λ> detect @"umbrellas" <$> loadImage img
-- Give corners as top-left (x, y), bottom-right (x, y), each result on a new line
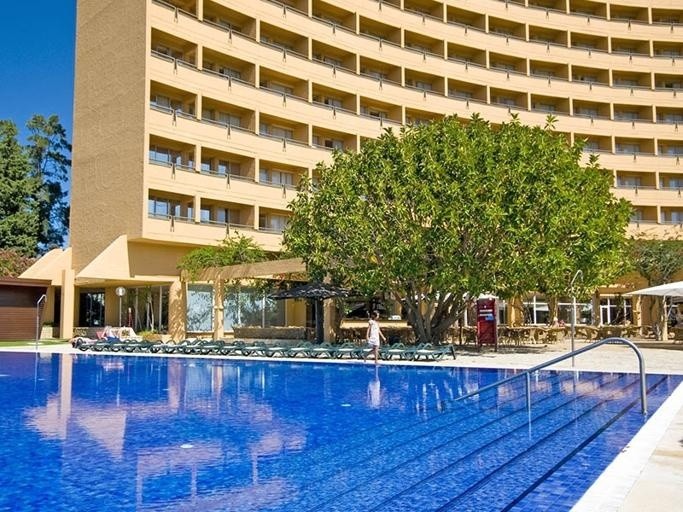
top-left (626, 281), bottom-right (683, 297)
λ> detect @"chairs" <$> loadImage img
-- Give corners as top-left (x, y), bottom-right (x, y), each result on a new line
top-left (457, 321), bottom-right (683, 349)
top-left (75, 336), bottom-right (457, 362)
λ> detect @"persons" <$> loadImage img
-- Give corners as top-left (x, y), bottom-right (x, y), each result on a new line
top-left (364, 310), bottom-right (387, 363)
top-left (366, 365), bottom-right (385, 416)
